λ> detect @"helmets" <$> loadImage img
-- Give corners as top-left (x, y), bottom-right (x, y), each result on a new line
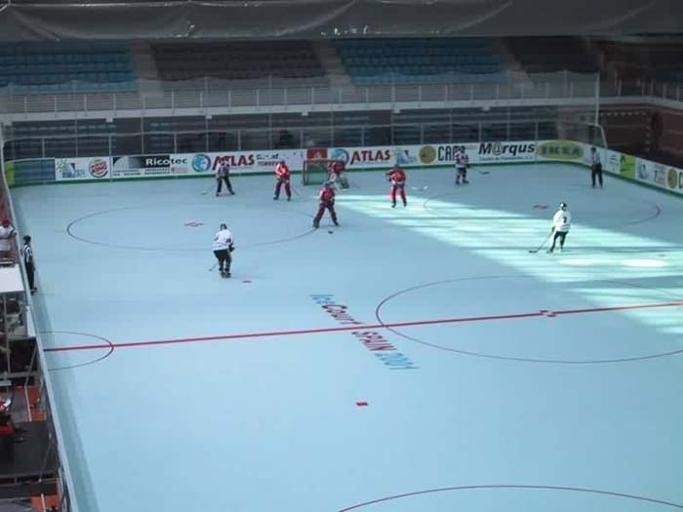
top-left (560, 203), bottom-right (569, 210)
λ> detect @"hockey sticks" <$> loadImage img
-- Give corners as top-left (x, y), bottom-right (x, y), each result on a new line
top-left (529, 232), bottom-right (553, 253)
top-left (208, 262), bottom-right (219, 272)
top-left (471, 167), bottom-right (490, 175)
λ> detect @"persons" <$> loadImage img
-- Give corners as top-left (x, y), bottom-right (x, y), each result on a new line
top-left (546, 202), bottom-right (571, 253)
top-left (213, 223), bottom-right (233, 277)
top-left (386, 163), bottom-right (408, 210)
top-left (588, 146), bottom-right (604, 189)
top-left (0, 217), bottom-right (16, 263)
top-left (214, 157), bottom-right (235, 198)
top-left (326, 160), bottom-right (348, 188)
top-left (0, 398), bottom-right (29, 446)
top-left (273, 159), bottom-right (293, 200)
top-left (20, 233), bottom-right (38, 298)
top-left (312, 179), bottom-right (338, 228)
top-left (450, 144), bottom-right (470, 185)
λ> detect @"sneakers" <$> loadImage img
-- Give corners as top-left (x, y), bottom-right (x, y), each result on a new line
top-left (221, 272), bottom-right (230, 278)
top-left (216, 191), bottom-right (235, 197)
top-left (455, 180), bottom-right (469, 184)
top-left (313, 221), bottom-right (338, 228)
top-left (391, 203), bottom-right (406, 207)
top-left (273, 197), bottom-right (290, 201)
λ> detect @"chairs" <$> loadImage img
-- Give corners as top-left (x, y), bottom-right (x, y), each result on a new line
top-left (1, 34), bottom-right (683, 161)
